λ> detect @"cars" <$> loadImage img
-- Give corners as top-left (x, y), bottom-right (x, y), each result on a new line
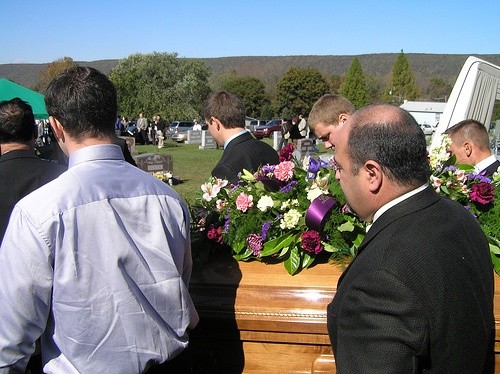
top-left (164, 121), bottom-right (193, 142)
top-left (249, 118), bottom-right (290, 140)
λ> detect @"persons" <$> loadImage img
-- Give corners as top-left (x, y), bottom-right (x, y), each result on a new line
top-left (327, 105), bottom-right (495, 374)
top-left (308, 94), bottom-right (356, 152)
top-left (442, 119), bottom-right (500, 180)
top-left (0, 90), bottom-right (308, 246)
top-left (0, 66), bottom-right (199, 374)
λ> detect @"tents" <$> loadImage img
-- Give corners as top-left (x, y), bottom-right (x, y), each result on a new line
top-left (0, 78), bottom-right (49, 119)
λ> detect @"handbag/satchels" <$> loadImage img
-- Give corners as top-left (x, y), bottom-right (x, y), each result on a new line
top-left (284, 132), bottom-right (291, 139)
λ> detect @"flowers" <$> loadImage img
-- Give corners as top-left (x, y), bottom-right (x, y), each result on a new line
top-left (186, 133), bottom-right (500, 276)
top-left (153, 171), bottom-right (172, 181)
